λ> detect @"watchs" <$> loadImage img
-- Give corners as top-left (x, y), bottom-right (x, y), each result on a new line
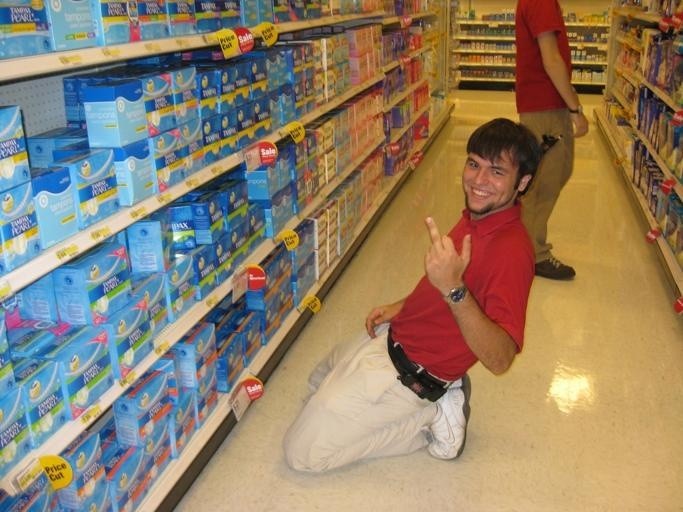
top-left (438, 284), bottom-right (471, 306)
top-left (569, 103), bottom-right (586, 115)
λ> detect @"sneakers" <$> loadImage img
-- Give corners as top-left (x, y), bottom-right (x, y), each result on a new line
top-left (429, 379), bottom-right (467, 462)
top-left (534, 254), bottom-right (576, 282)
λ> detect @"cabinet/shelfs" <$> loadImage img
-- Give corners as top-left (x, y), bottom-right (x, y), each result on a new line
top-left (0, 9), bottom-right (455, 512)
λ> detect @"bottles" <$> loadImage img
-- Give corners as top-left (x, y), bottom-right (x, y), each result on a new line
top-left (452, 8), bottom-right (516, 78)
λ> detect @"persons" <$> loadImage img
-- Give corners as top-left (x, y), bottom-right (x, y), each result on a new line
top-left (282, 119), bottom-right (541, 473)
top-left (514, 0), bottom-right (589, 281)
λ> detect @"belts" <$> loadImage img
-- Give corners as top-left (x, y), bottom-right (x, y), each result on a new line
top-left (387, 329), bottom-right (446, 402)
